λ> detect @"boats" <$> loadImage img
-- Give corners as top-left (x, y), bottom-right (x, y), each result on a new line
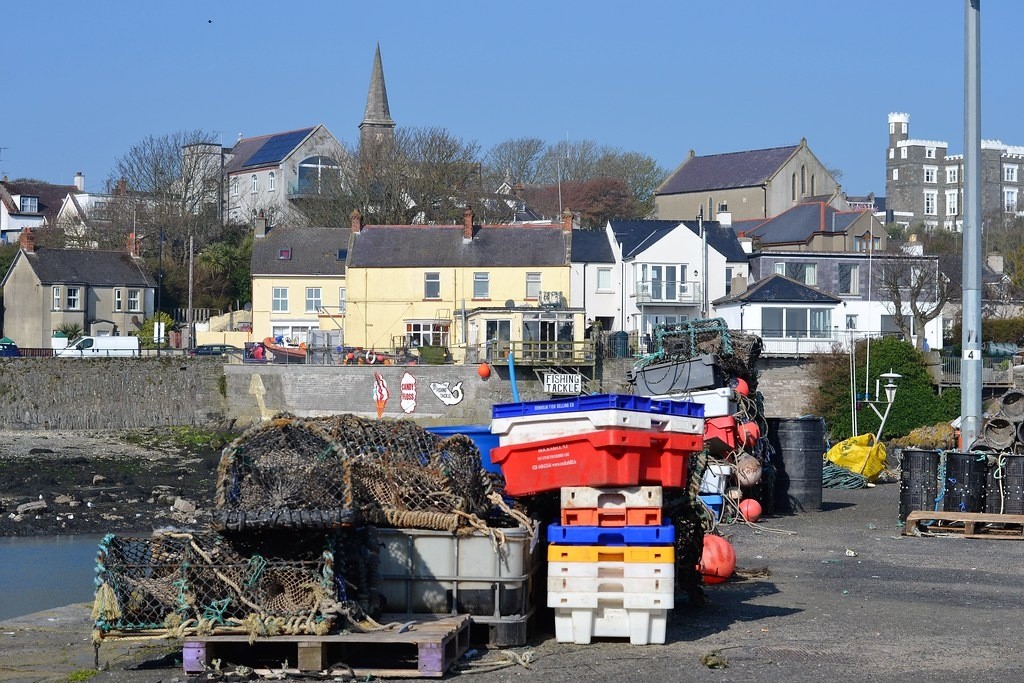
top-left (264, 334), bottom-right (308, 359)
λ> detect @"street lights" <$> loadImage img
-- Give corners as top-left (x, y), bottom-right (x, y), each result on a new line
top-left (157, 226), bottom-right (168, 357)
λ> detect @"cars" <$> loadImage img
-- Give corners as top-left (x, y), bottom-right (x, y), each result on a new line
top-left (189, 343), bottom-right (243, 356)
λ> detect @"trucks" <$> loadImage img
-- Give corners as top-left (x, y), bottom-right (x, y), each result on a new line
top-left (56, 336), bottom-right (141, 358)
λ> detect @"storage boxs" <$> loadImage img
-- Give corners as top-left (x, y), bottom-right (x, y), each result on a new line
top-left (627, 353), bottom-right (746, 527)
top-left (490, 394), bottom-right (704, 646)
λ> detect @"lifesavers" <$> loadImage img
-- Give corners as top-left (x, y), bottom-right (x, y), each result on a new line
top-left (366, 351), bottom-right (376, 364)
